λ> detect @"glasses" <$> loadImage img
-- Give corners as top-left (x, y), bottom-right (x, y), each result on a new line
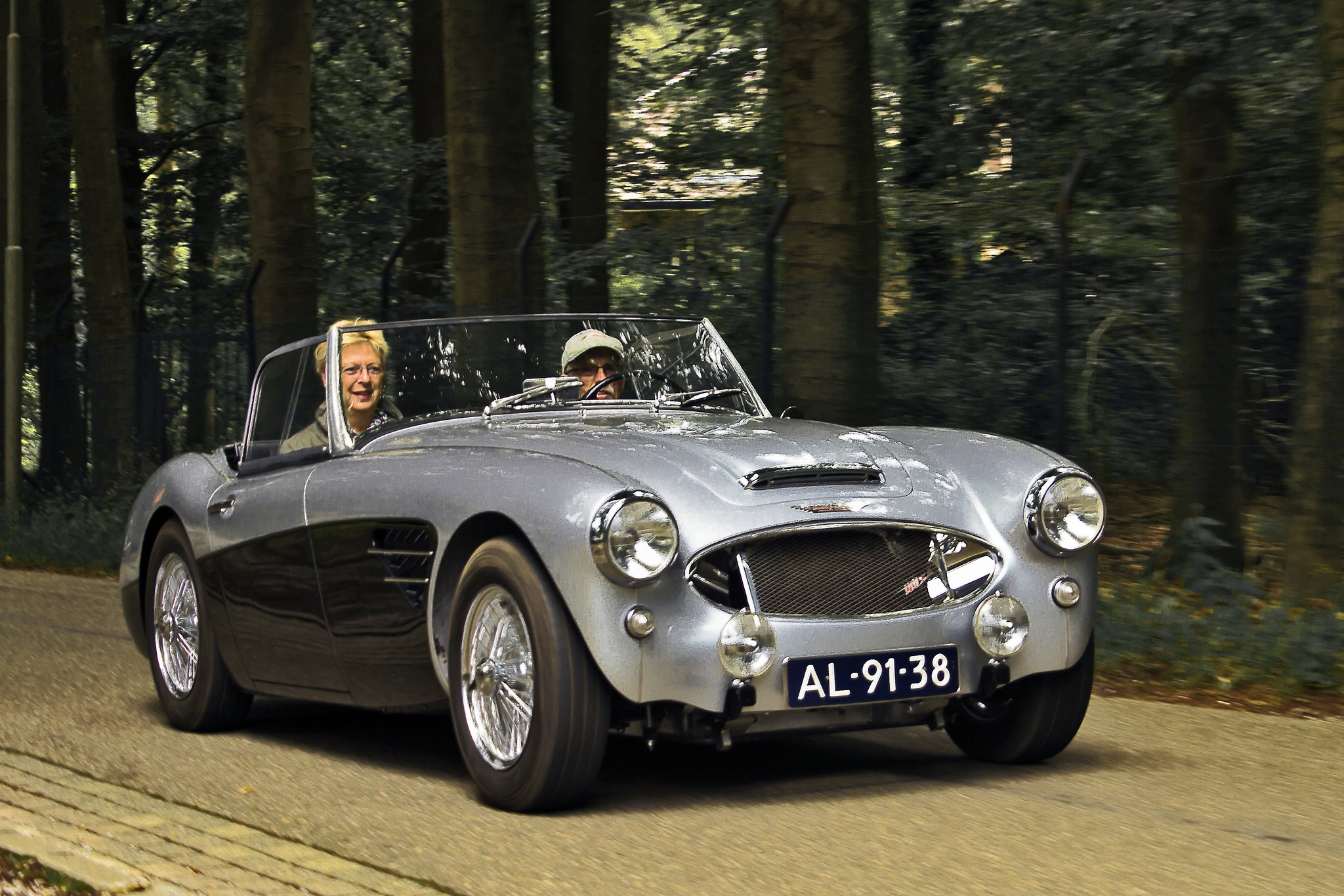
top-left (564, 363), bottom-right (624, 376)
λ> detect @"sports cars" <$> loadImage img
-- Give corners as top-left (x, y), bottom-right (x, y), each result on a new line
top-left (118, 312), bottom-right (1108, 815)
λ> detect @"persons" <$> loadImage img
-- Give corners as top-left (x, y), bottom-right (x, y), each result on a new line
top-left (560, 329), bottom-right (626, 399)
top-left (280, 319), bottom-right (403, 454)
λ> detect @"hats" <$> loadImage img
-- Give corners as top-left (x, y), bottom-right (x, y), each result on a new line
top-left (562, 329), bottom-right (625, 374)
